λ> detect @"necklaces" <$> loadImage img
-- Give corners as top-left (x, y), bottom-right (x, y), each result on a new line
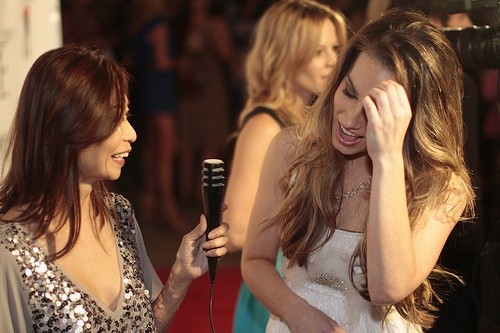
top-left (335, 176), bottom-right (372, 199)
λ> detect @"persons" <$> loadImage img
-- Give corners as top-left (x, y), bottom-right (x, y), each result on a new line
top-left (130, 16), bottom-right (192, 233)
top-left (0, 46), bottom-right (230, 333)
top-left (240, 9), bottom-right (475, 333)
top-left (365, 0), bottom-right (472, 28)
top-left (219, 0), bottom-right (350, 333)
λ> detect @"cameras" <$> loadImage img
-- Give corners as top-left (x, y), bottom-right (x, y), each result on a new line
top-left (442, 24), bottom-right (499, 68)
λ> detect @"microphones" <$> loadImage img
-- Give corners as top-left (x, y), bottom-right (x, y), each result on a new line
top-left (202, 159), bottom-right (226, 279)
